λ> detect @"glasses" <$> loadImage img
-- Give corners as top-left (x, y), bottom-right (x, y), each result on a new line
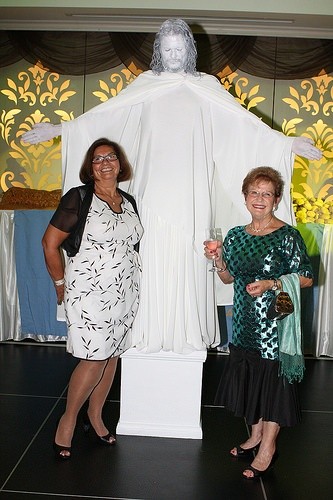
top-left (91, 154), bottom-right (120, 164)
top-left (246, 189), bottom-right (276, 198)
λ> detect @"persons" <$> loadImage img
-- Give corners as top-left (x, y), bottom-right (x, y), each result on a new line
top-left (203, 167), bottom-right (314, 478)
top-left (21, 19), bottom-right (323, 348)
top-left (41, 138), bottom-right (144, 458)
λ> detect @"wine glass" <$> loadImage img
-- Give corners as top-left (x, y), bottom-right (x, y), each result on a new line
top-left (206, 228), bottom-right (222, 272)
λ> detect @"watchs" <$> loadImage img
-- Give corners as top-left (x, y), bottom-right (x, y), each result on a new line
top-left (271, 280), bottom-right (278, 290)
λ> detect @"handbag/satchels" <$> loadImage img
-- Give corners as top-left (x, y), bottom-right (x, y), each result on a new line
top-left (267, 278), bottom-right (294, 320)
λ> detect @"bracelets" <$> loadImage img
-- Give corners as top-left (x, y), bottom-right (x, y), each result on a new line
top-left (216, 262), bottom-right (227, 272)
top-left (54, 278), bottom-right (66, 285)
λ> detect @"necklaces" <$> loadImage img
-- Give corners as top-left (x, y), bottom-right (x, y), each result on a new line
top-left (102, 191), bottom-right (120, 205)
top-left (250, 217), bottom-right (273, 233)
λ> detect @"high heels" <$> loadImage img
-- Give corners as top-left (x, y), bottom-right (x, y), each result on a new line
top-left (53, 415), bottom-right (76, 460)
top-left (228, 437), bottom-right (262, 458)
top-left (82, 411), bottom-right (117, 447)
top-left (240, 445), bottom-right (281, 479)
